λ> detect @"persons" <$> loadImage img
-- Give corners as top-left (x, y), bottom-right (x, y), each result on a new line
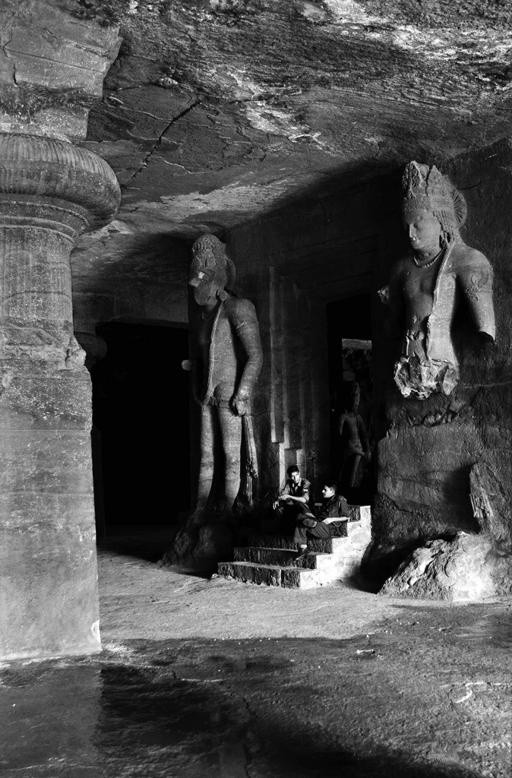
top-left (272, 465), bottom-right (310, 514)
top-left (293, 480), bottom-right (350, 560)
top-left (374, 160), bottom-right (496, 398)
top-left (338, 383), bottom-right (371, 488)
top-left (185, 234), bottom-right (262, 522)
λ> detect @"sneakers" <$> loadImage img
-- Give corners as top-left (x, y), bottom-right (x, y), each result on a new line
top-left (295, 547), bottom-right (311, 561)
top-left (303, 517), bottom-right (317, 528)
